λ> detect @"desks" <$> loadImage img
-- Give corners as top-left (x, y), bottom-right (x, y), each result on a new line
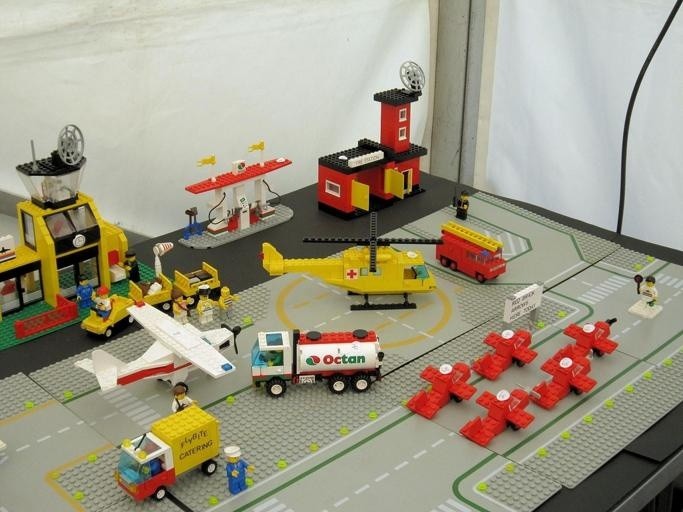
top-left (0, 146), bottom-right (683, 512)
